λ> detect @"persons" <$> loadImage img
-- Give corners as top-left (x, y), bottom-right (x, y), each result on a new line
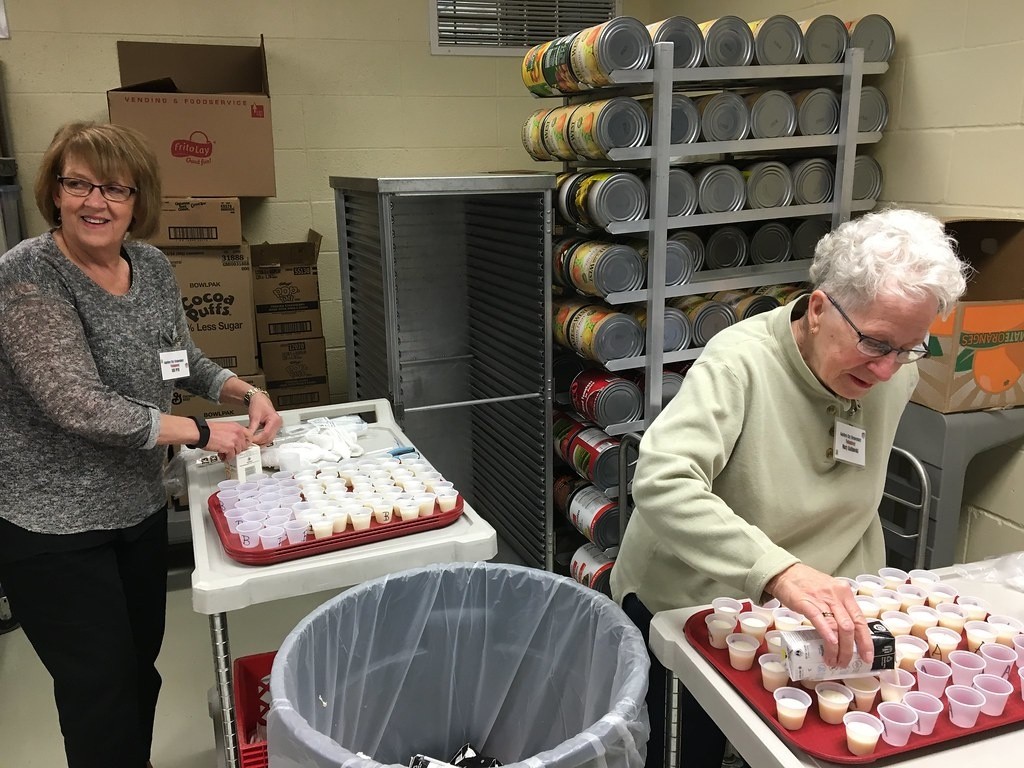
top-left (0, 119), bottom-right (284, 768)
top-left (608, 206), bottom-right (976, 768)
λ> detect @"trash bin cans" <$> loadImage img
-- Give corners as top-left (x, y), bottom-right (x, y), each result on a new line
top-left (267, 561), bottom-right (655, 768)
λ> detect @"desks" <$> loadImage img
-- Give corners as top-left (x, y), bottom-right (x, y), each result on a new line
top-left (649, 556), bottom-right (1022, 768)
top-left (188, 404), bottom-right (497, 768)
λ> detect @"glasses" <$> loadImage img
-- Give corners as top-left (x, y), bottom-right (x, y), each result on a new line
top-left (56, 172), bottom-right (139, 203)
top-left (818, 288), bottom-right (931, 364)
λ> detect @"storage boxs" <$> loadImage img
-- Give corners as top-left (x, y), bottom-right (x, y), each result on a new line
top-left (104, 35), bottom-right (331, 512)
top-left (908, 216), bottom-right (1024, 414)
top-left (232, 649), bottom-right (278, 768)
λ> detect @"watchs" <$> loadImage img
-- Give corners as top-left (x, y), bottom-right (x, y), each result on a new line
top-left (244, 386), bottom-right (271, 406)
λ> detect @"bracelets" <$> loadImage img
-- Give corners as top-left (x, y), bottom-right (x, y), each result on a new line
top-left (185, 415), bottom-right (211, 449)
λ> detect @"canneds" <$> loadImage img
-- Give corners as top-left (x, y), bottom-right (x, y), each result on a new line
top-left (520, 13), bottom-right (897, 598)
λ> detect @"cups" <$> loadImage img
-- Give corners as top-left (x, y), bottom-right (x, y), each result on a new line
top-left (925, 626), bottom-right (963, 663)
top-left (948, 649), bottom-right (986, 687)
top-left (1013, 634), bottom-right (1024, 668)
top-left (980, 643), bottom-right (1019, 682)
top-left (773, 687), bottom-right (812, 730)
top-left (943, 685), bottom-right (986, 728)
top-left (844, 709), bottom-right (884, 757)
top-left (1018, 667), bottom-right (1024, 701)
top-left (903, 691), bottom-right (944, 736)
top-left (758, 653), bottom-right (790, 692)
top-left (988, 615), bottom-right (1024, 650)
top-left (217, 471), bottom-right (311, 549)
top-left (815, 681), bottom-right (854, 724)
top-left (964, 620), bottom-right (999, 656)
top-left (835, 568), bottom-right (989, 643)
top-left (972, 673), bottom-right (1013, 716)
top-left (842, 677), bottom-right (882, 713)
top-left (295, 453), bottom-right (459, 539)
top-left (877, 701), bottom-right (919, 748)
top-left (914, 658), bottom-right (952, 698)
top-left (880, 667), bottom-right (917, 704)
top-left (705, 597), bottom-right (815, 671)
top-left (895, 635), bottom-right (930, 673)
top-left (801, 680), bottom-right (822, 690)
